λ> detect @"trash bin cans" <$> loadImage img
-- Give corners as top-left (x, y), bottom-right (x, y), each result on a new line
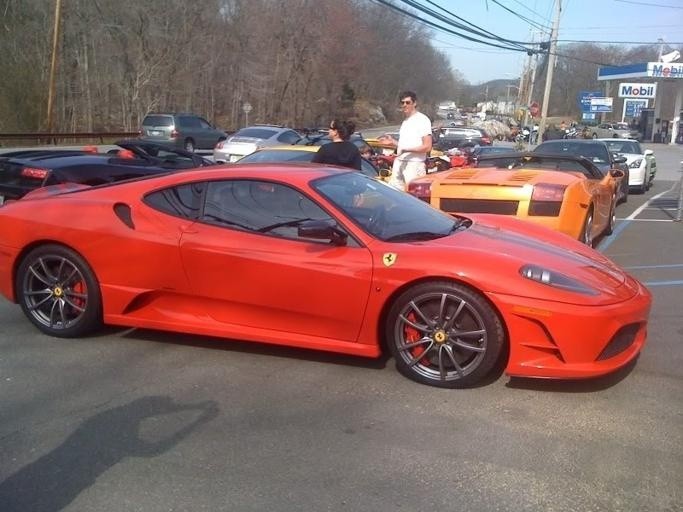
top-left (654, 134), bottom-right (662, 142)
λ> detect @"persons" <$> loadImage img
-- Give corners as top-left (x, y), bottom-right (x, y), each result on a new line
top-left (310, 117), bottom-right (361, 170)
top-left (358, 145), bottom-right (373, 158)
top-left (385, 91), bottom-right (433, 196)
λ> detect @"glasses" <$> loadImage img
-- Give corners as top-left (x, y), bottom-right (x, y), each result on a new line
top-left (401, 101), bottom-right (411, 104)
top-left (329, 126), bottom-right (335, 130)
